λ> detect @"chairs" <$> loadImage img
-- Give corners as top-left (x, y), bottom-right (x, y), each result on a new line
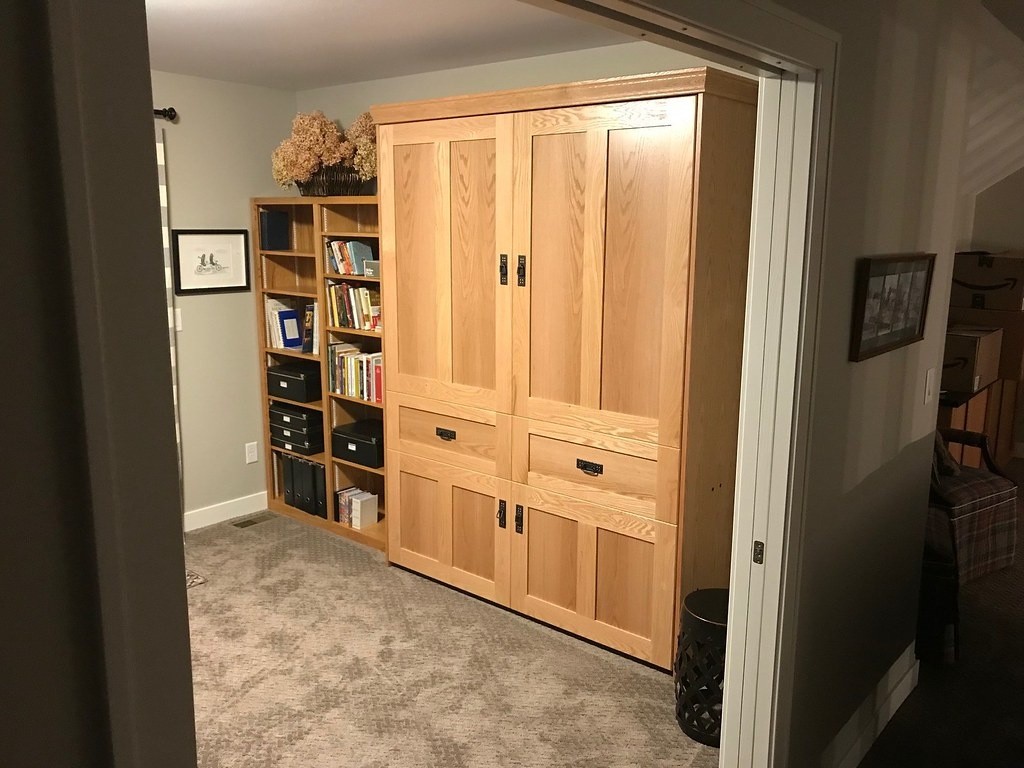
top-left (924, 402), bottom-right (1017, 583)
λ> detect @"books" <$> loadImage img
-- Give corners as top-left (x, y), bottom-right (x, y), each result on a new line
top-left (260, 212), bottom-right (289, 250)
top-left (327, 340), bottom-right (383, 403)
top-left (334, 486), bottom-right (378, 529)
top-left (265, 294), bottom-right (319, 355)
top-left (326, 282), bottom-right (380, 331)
top-left (326, 240), bottom-right (373, 276)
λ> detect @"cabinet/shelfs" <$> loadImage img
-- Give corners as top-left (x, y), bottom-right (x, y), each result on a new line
top-left (249, 195), bottom-right (387, 552)
top-left (369, 67), bottom-right (757, 675)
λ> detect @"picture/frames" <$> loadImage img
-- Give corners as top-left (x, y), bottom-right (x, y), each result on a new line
top-left (850, 252), bottom-right (939, 363)
top-left (172, 228), bottom-right (251, 297)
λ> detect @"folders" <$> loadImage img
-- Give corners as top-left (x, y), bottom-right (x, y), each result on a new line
top-left (282, 453), bottom-right (294, 507)
top-left (315, 464), bottom-right (327, 520)
top-left (292, 457), bottom-right (303, 511)
top-left (302, 460), bottom-right (316, 516)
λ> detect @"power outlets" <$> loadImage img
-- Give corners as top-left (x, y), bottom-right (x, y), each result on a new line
top-left (245, 442), bottom-right (258, 464)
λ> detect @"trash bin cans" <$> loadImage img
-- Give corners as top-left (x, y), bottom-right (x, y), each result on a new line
top-left (673, 587), bottom-right (730, 747)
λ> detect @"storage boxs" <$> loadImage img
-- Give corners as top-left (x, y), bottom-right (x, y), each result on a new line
top-left (267, 363), bottom-right (321, 404)
top-left (333, 419), bottom-right (384, 469)
top-left (941, 248), bottom-right (1024, 393)
top-left (268, 404), bottom-right (325, 518)
